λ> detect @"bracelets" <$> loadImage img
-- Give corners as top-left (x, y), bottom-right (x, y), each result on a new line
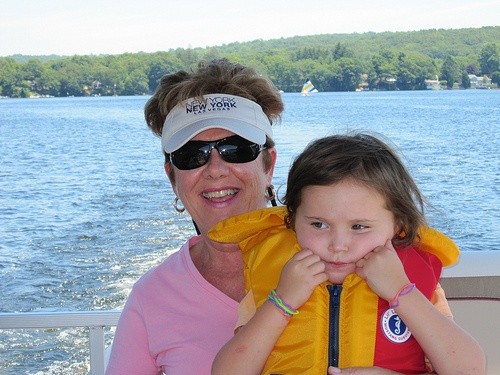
top-left (387, 282), bottom-right (417, 309)
top-left (263, 288), bottom-right (304, 322)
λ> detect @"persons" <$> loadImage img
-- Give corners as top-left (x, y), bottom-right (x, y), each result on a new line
top-left (207, 135), bottom-right (486, 375)
top-left (106, 58), bottom-right (284, 375)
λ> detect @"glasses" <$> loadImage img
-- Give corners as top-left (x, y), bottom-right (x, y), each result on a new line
top-left (168, 135), bottom-right (261, 171)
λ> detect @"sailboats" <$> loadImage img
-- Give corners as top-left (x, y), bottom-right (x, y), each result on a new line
top-left (301, 78), bottom-right (319, 95)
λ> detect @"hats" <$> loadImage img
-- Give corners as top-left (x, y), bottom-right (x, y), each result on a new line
top-left (162, 94), bottom-right (273, 154)
